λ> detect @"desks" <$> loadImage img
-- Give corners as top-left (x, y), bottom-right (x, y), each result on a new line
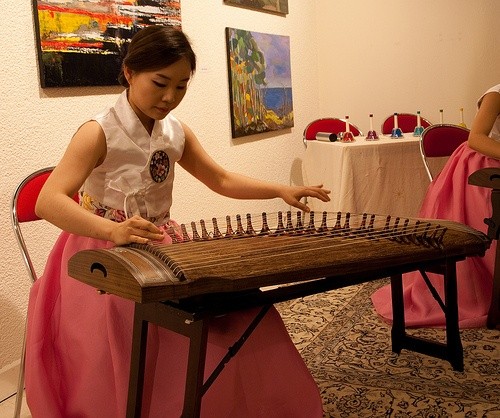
top-left (302, 132), bottom-right (452, 221)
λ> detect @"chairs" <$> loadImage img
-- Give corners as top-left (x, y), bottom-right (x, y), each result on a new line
top-left (302, 116), bottom-right (363, 148)
top-left (11, 165), bottom-right (80, 418)
top-left (420, 123), bottom-right (471, 181)
top-left (381, 112), bottom-right (433, 134)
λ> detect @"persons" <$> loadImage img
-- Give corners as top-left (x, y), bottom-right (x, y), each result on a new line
top-left (25, 24), bottom-right (330, 418)
top-left (371, 83), bottom-right (500, 330)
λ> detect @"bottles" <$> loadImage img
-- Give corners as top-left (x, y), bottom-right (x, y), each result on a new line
top-left (316, 132), bottom-right (337, 142)
